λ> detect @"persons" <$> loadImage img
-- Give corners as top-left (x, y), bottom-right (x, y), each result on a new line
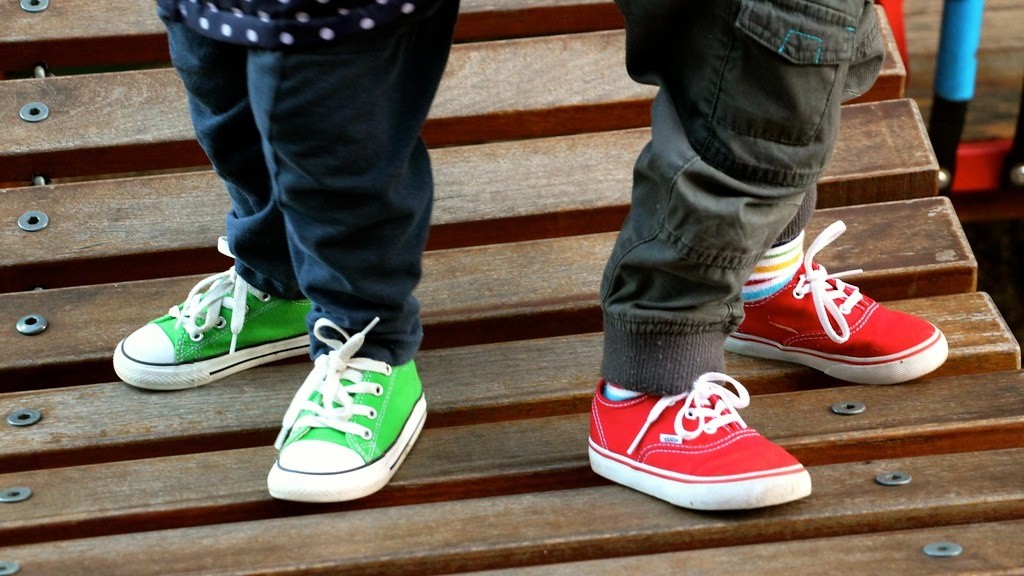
top-left (113, 0), bottom-right (463, 503)
top-left (588, 0), bottom-right (949, 511)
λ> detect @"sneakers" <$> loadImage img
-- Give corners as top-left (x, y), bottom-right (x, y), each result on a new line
top-left (113, 236), bottom-right (313, 390)
top-left (588, 371), bottom-right (812, 511)
top-left (724, 220), bottom-right (949, 384)
top-left (266, 317), bottom-right (427, 502)
